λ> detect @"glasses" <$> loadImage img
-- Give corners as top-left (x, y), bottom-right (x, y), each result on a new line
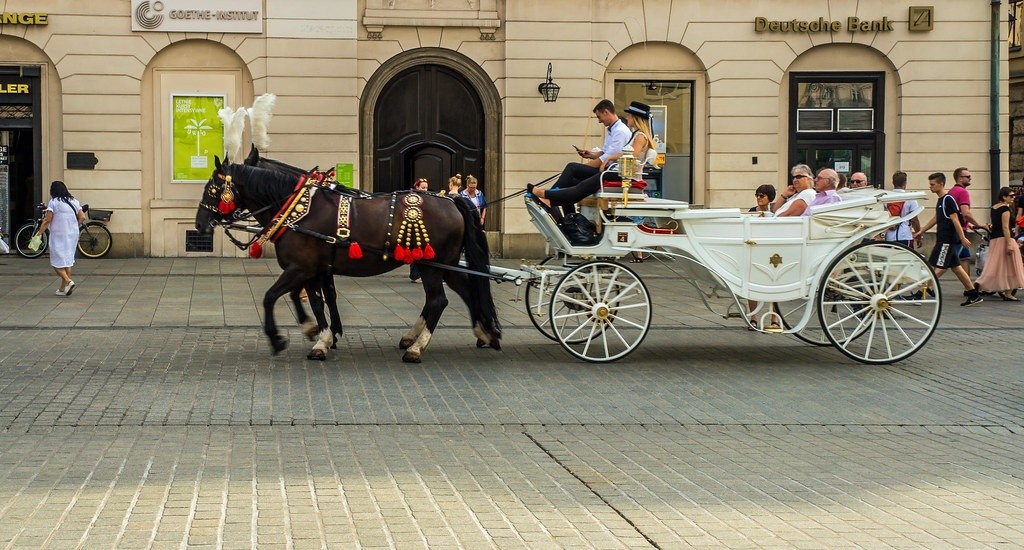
top-left (1004, 194), bottom-right (1015, 198)
top-left (467, 185), bottom-right (476, 189)
top-left (419, 179), bottom-right (427, 182)
top-left (791, 175), bottom-right (807, 180)
top-left (814, 175), bottom-right (829, 181)
top-left (754, 194), bottom-right (765, 198)
top-left (960, 175), bottom-right (971, 178)
top-left (851, 179), bottom-right (864, 184)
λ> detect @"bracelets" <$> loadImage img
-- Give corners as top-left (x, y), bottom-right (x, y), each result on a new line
top-left (781, 193), bottom-right (787, 200)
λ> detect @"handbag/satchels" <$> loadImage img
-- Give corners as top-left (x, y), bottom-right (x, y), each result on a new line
top-left (975, 243), bottom-right (990, 271)
top-left (558, 212), bottom-right (600, 245)
top-left (28, 231), bottom-right (42, 252)
top-left (0, 237), bottom-right (9, 254)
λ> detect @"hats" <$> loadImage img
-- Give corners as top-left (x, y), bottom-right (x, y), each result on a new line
top-left (624, 100), bottom-right (650, 119)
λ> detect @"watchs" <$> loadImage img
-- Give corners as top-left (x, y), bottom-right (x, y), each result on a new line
top-left (879, 232), bottom-right (885, 238)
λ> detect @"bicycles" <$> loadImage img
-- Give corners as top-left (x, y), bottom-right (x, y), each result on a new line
top-left (14, 203), bottom-right (113, 259)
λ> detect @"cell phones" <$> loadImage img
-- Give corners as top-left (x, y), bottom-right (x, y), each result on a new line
top-left (572, 145), bottom-right (580, 152)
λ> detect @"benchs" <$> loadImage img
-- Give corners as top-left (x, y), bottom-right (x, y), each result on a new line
top-left (587, 149), bottom-right (658, 192)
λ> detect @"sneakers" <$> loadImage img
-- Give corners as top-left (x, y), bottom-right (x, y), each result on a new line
top-left (960, 293), bottom-right (983, 307)
top-left (55, 280), bottom-right (75, 296)
top-left (901, 291), bottom-right (922, 305)
top-left (925, 286), bottom-right (936, 297)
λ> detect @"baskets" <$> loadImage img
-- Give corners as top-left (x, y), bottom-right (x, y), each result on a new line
top-left (88, 209), bottom-right (113, 221)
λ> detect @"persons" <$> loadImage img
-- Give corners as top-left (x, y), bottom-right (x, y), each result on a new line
top-left (902, 173), bottom-right (983, 306)
top-left (831, 172), bottom-right (896, 320)
top-left (799, 169), bottom-right (842, 217)
top-left (442, 174), bottom-right (462, 284)
top-left (36, 181), bottom-right (86, 296)
top-left (748, 185), bottom-right (781, 331)
top-left (527, 99), bottom-right (656, 217)
top-left (973, 187), bottom-right (1024, 302)
top-left (772, 163), bottom-right (817, 217)
top-left (408, 177), bottom-right (428, 283)
top-left (947, 167), bottom-right (992, 297)
top-left (459, 174), bottom-right (488, 225)
top-left (883, 171), bottom-right (922, 300)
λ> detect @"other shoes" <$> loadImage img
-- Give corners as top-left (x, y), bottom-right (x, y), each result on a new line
top-left (630, 256), bottom-right (643, 263)
top-left (532, 195), bottom-right (552, 213)
top-left (1004, 294), bottom-right (1021, 301)
top-left (973, 282), bottom-right (980, 292)
top-left (889, 289), bottom-right (905, 301)
top-left (527, 183), bottom-right (535, 194)
top-left (410, 277), bottom-right (423, 283)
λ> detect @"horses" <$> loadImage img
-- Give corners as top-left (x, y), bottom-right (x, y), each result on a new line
top-left (195, 143), bottom-right (504, 363)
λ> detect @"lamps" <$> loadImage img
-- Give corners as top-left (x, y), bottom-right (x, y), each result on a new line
top-left (538, 61), bottom-right (560, 103)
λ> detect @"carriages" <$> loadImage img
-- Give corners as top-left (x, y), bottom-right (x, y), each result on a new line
top-left (194, 148), bottom-right (942, 366)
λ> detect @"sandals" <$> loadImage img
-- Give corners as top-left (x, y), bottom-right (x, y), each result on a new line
top-left (770, 322), bottom-right (779, 328)
top-left (748, 320), bottom-right (757, 331)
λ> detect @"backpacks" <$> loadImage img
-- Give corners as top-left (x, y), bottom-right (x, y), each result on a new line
top-left (885, 201), bottom-right (904, 231)
